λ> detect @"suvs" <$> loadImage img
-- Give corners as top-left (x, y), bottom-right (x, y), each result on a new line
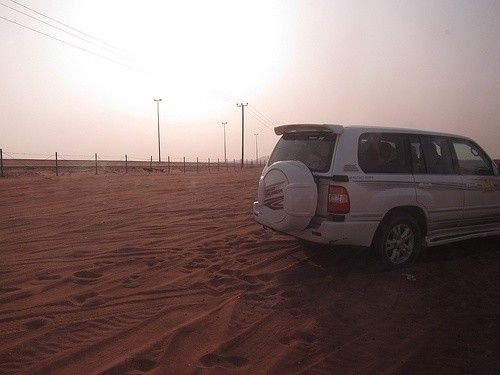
top-left (252, 123), bottom-right (500, 272)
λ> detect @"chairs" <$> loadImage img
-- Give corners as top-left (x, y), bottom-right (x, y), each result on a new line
top-left (418, 143), bottom-right (447, 174)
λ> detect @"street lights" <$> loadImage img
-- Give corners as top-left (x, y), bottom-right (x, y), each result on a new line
top-left (154, 98), bottom-right (162, 162)
top-left (222, 121), bottom-right (227, 163)
top-left (254, 134), bottom-right (259, 164)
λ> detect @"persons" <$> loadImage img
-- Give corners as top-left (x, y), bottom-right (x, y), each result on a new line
top-left (419, 142), bottom-right (449, 174)
